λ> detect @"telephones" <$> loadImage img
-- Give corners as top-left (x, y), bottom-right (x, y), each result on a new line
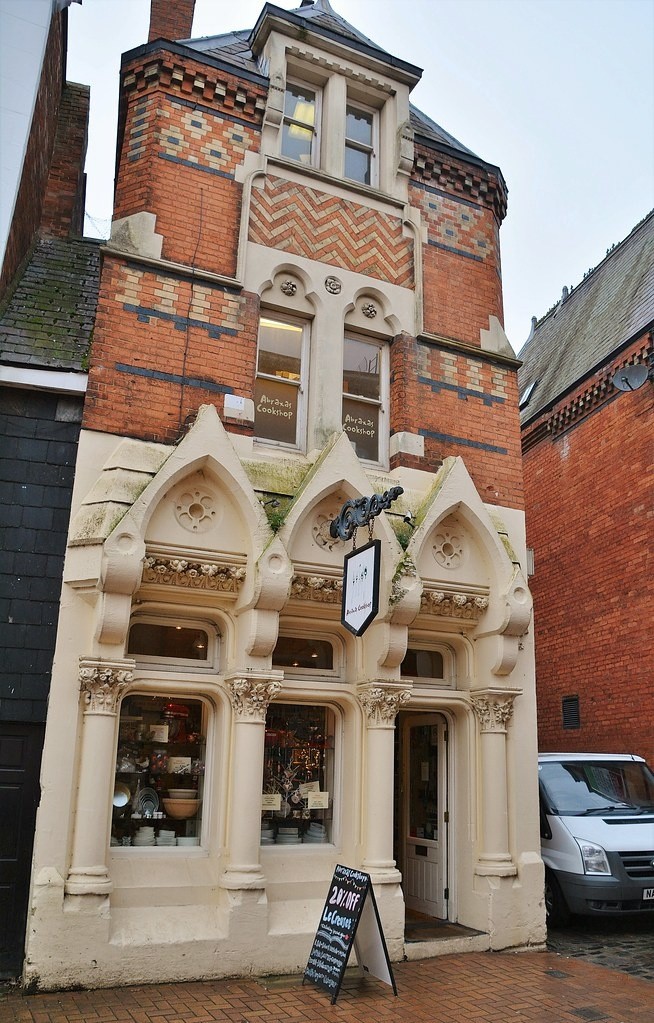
top-left (304, 862), bottom-right (396, 997)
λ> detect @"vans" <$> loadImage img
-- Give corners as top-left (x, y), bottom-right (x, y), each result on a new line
top-left (540, 752), bottom-right (654, 927)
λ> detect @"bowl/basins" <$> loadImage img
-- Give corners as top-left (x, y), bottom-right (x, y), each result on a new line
top-left (111, 780), bottom-right (329, 847)
